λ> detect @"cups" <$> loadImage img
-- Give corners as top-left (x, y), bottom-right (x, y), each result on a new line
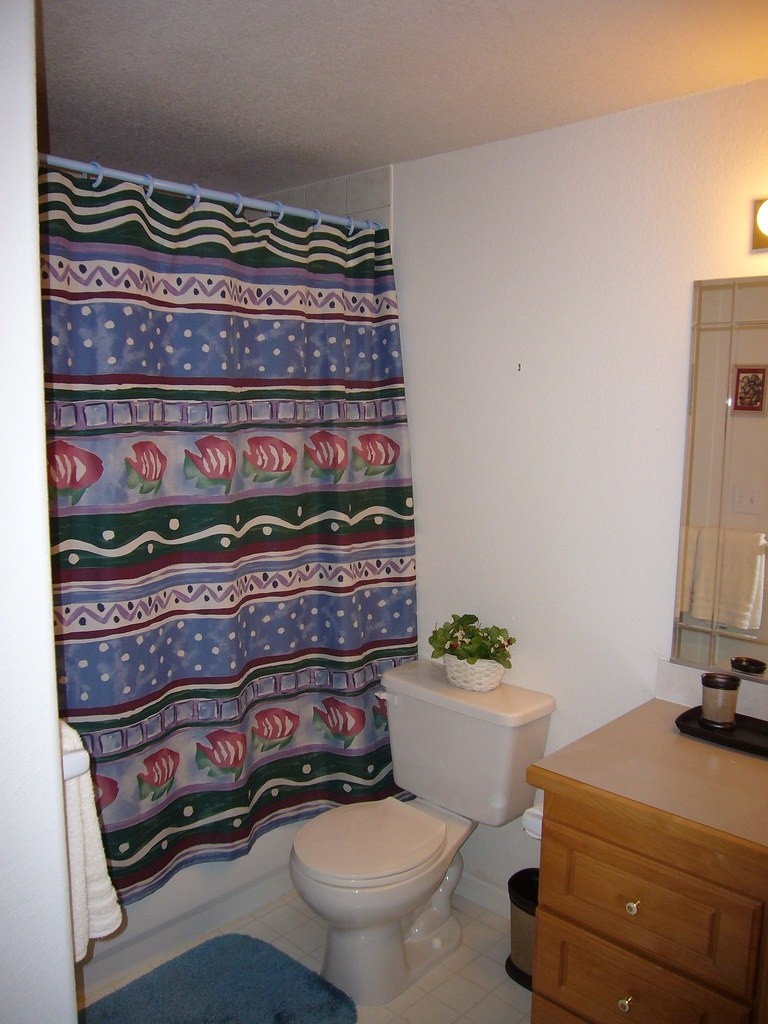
top-left (697, 673), bottom-right (741, 737)
top-left (731, 656), bottom-right (768, 678)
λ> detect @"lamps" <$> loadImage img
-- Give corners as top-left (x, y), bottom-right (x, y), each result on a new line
top-left (751, 198), bottom-right (768, 249)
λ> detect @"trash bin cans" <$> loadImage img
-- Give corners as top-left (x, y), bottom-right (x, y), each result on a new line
top-left (505, 867), bottom-right (540, 993)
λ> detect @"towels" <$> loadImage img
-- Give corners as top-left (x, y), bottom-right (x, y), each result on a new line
top-left (57, 714), bottom-right (125, 965)
top-left (673, 525), bottom-right (767, 631)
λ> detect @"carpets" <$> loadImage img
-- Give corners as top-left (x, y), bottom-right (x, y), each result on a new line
top-left (78, 934), bottom-right (357, 1024)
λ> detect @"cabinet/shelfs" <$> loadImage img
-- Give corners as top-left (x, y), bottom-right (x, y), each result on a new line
top-left (525, 701), bottom-right (768, 1024)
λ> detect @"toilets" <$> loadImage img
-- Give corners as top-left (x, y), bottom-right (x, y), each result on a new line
top-left (287, 657), bottom-right (557, 1009)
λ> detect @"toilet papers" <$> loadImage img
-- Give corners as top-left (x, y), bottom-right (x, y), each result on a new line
top-left (521, 801), bottom-right (544, 840)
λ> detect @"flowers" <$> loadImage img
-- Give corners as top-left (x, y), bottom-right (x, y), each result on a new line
top-left (428, 614), bottom-right (516, 669)
